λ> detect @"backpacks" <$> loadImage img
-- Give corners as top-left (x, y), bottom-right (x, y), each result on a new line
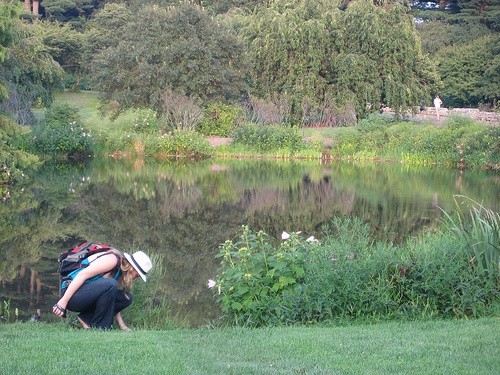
top-left (57, 242), bottom-right (113, 277)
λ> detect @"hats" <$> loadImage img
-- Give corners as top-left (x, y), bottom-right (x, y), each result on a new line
top-left (124, 250), bottom-right (152, 282)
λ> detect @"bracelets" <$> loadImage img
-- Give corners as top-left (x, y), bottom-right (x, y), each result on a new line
top-left (54, 302), bottom-right (66, 313)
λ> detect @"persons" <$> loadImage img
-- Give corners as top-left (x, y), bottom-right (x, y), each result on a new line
top-left (53, 247), bottom-right (153, 334)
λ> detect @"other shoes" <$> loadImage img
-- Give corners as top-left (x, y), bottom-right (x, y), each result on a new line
top-left (68, 319), bottom-right (90, 331)
